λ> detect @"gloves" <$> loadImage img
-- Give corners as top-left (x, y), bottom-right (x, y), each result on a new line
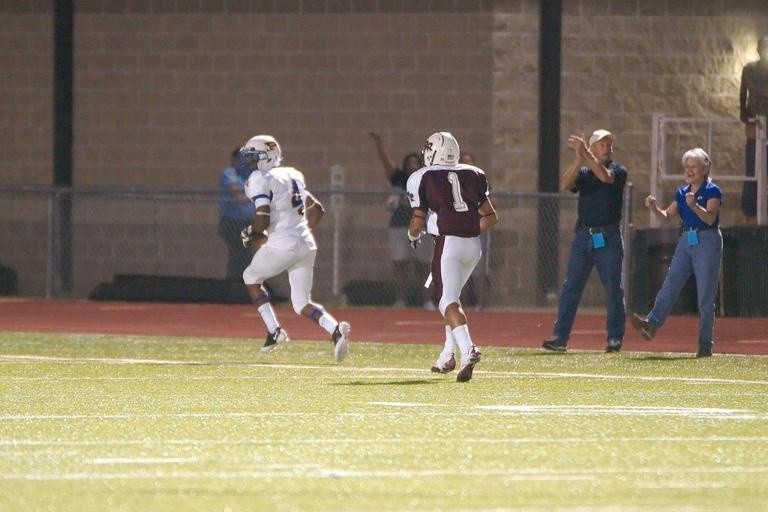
top-left (407, 230), bottom-right (423, 251)
top-left (238, 224), bottom-right (256, 250)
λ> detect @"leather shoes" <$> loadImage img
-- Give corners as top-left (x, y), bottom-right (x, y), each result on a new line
top-left (630, 312), bottom-right (656, 342)
top-left (542, 337), bottom-right (567, 353)
top-left (605, 344), bottom-right (621, 354)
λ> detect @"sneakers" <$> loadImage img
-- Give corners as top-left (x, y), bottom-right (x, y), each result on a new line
top-left (456, 345), bottom-right (481, 383)
top-left (430, 353), bottom-right (456, 375)
top-left (261, 327), bottom-right (288, 352)
top-left (331, 320), bottom-right (350, 363)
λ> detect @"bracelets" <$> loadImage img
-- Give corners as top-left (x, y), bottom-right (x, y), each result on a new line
top-left (690, 203), bottom-right (698, 212)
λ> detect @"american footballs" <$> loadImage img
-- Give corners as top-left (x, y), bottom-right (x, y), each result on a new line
top-left (250, 230), bottom-right (268, 252)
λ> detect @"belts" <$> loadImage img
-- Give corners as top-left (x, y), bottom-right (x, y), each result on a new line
top-left (683, 226), bottom-right (711, 231)
top-left (578, 222), bottom-right (617, 235)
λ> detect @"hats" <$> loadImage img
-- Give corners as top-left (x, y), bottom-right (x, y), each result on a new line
top-left (589, 129), bottom-right (615, 148)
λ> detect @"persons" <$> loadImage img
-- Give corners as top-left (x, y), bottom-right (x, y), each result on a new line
top-left (408, 131), bottom-right (499, 382)
top-left (543, 129), bottom-right (627, 352)
top-left (236, 135), bottom-right (351, 363)
top-left (218, 149), bottom-right (290, 306)
top-left (454, 152), bottom-right (491, 312)
top-left (629, 148), bottom-right (725, 358)
top-left (736, 36), bottom-right (768, 225)
top-left (368, 130), bottom-right (436, 310)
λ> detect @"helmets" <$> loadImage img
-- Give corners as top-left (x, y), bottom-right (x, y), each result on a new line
top-left (421, 131), bottom-right (460, 166)
top-left (237, 134), bottom-right (282, 179)
